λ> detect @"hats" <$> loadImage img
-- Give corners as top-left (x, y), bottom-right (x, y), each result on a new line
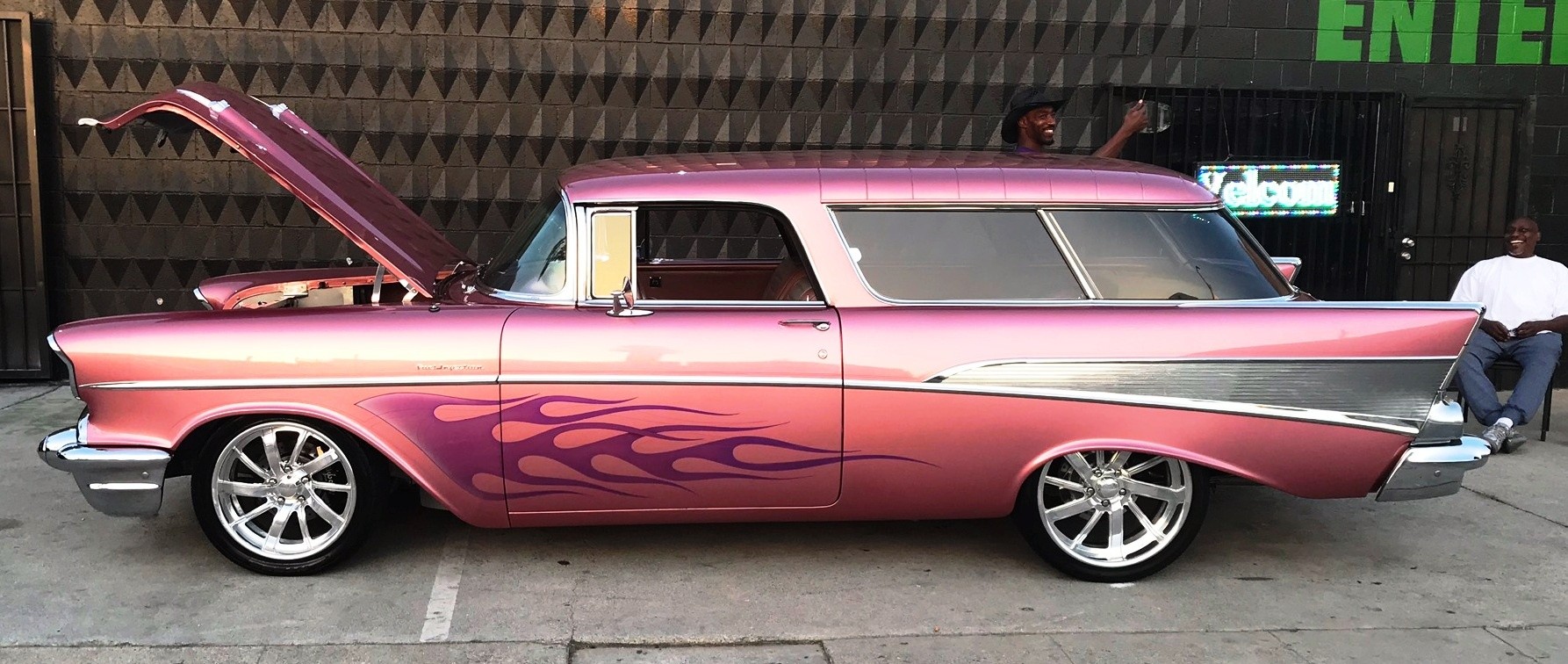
top-left (1001, 85), bottom-right (1074, 144)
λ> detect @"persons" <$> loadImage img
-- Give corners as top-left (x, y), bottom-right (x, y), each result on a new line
top-left (1449, 217), bottom-right (1568, 452)
top-left (1010, 87), bottom-right (1149, 158)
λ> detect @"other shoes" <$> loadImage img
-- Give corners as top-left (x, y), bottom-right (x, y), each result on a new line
top-left (1480, 423), bottom-right (1511, 453)
top-left (1504, 430), bottom-right (1528, 455)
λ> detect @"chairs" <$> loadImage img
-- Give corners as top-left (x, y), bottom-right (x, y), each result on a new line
top-left (763, 258), bottom-right (815, 302)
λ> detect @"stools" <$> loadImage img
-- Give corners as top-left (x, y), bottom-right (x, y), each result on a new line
top-left (1456, 359), bottom-right (1552, 441)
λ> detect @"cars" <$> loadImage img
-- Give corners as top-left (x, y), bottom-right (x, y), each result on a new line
top-left (34, 81), bottom-right (1497, 583)
